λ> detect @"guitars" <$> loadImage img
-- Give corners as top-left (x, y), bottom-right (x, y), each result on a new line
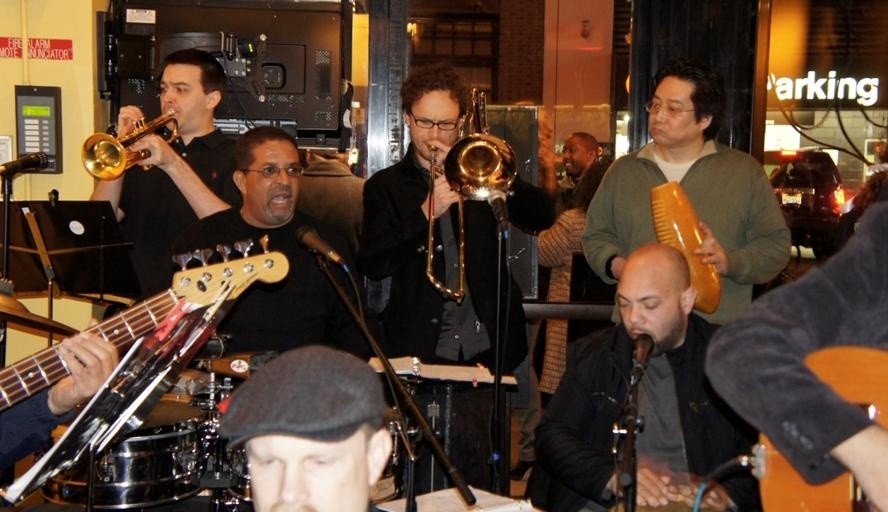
top-left (758, 343), bottom-right (888, 511)
top-left (1, 236), bottom-right (291, 411)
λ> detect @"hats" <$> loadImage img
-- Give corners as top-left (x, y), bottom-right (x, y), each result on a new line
top-left (218, 345), bottom-right (386, 449)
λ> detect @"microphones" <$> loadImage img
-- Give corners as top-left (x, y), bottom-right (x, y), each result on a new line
top-left (629, 333), bottom-right (654, 383)
top-left (295, 225), bottom-right (343, 265)
top-left (0, 152), bottom-right (48, 178)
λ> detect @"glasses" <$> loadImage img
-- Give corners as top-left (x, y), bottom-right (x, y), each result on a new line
top-left (404, 108), bottom-right (466, 133)
top-left (644, 101), bottom-right (699, 119)
top-left (237, 164), bottom-right (304, 180)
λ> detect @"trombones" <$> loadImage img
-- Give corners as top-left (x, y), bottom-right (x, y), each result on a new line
top-left (427, 86), bottom-right (519, 302)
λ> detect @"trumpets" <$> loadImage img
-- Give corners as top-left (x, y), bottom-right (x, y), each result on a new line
top-left (83, 109), bottom-right (180, 182)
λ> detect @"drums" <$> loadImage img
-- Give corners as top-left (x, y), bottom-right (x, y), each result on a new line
top-left (31, 401), bottom-right (207, 511)
top-left (226, 406), bottom-right (398, 504)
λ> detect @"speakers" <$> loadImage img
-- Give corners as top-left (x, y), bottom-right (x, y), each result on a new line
top-left (464, 104), bottom-right (539, 301)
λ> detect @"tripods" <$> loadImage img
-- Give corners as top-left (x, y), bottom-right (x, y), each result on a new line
top-left (0, 199), bottom-right (141, 347)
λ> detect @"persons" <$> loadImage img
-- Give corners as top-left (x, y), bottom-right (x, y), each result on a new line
top-left (536, 159), bottom-right (603, 393)
top-left (0, 319), bottom-right (119, 469)
top-left (169, 127), bottom-right (367, 359)
top-left (362, 69), bottom-right (556, 380)
top-left (296, 145), bottom-right (365, 234)
top-left (215, 345), bottom-right (393, 511)
top-left (580, 57), bottom-right (793, 327)
top-left (538, 117), bottom-right (600, 195)
top-left (704, 201), bottom-right (888, 512)
top-left (534, 244), bottom-right (764, 512)
top-left (88, 48), bottom-right (242, 294)
